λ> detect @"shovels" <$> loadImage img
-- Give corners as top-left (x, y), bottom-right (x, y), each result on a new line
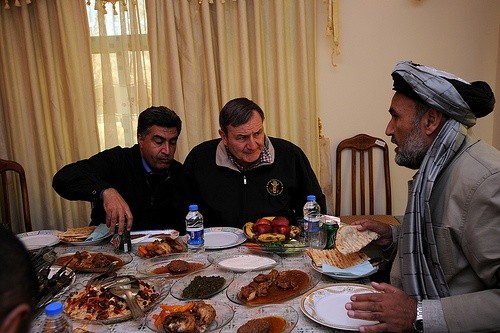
top-left (110, 275), bottom-right (146, 320)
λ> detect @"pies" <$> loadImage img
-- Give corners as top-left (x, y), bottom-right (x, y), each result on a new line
top-left (335, 224), bottom-right (380, 256)
top-left (308, 248), bottom-right (370, 267)
top-left (289, 225), bottom-right (300, 237)
top-left (262, 217), bottom-right (275, 221)
top-left (59, 226), bottom-right (98, 243)
top-left (258, 233), bottom-right (285, 242)
top-left (245, 222), bottom-right (256, 237)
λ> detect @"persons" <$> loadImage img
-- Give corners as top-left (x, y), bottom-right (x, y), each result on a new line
top-left (182, 98), bottom-right (327, 230)
top-left (0, 226), bottom-right (41, 333)
top-left (345, 61), bottom-right (500, 333)
top-left (52, 105), bottom-right (184, 235)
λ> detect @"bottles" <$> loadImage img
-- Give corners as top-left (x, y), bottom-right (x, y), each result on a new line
top-left (41, 302), bottom-right (74, 333)
top-left (185, 204), bottom-right (205, 261)
top-left (303, 195), bottom-right (320, 250)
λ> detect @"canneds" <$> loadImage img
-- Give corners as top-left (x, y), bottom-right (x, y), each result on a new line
top-left (321, 221), bottom-right (339, 249)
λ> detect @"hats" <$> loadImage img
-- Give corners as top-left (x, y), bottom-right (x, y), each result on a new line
top-left (390, 61), bottom-right (495, 128)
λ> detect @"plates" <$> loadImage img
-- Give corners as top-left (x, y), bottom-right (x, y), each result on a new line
top-left (145, 298), bottom-right (235, 333)
top-left (226, 264), bottom-right (321, 305)
top-left (218, 303), bottom-right (299, 333)
top-left (300, 283), bottom-right (385, 331)
top-left (62, 275), bottom-right (171, 325)
top-left (15, 229), bottom-right (64, 251)
top-left (136, 253), bottom-right (214, 278)
top-left (52, 244), bottom-right (132, 275)
top-left (32, 265), bottom-right (77, 311)
top-left (206, 248), bottom-right (281, 272)
top-left (310, 252), bottom-right (378, 280)
top-left (195, 227), bottom-right (247, 250)
top-left (170, 267), bottom-right (234, 299)
top-left (62, 230), bottom-right (112, 246)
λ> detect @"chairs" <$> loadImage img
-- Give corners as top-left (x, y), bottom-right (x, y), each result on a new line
top-left (335, 133), bottom-right (401, 225)
top-left (0, 158), bottom-right (33, 234)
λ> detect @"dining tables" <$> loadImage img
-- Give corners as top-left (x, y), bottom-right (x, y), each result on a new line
top-left (27, 236), bottom-right (378, 333)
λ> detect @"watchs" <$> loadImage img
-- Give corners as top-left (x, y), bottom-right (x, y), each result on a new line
top-left (413, 300), bottom-right (424, 333)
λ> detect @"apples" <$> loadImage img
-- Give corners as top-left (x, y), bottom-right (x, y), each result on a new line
top-left (254, 216), bottom-right (290, 236)
